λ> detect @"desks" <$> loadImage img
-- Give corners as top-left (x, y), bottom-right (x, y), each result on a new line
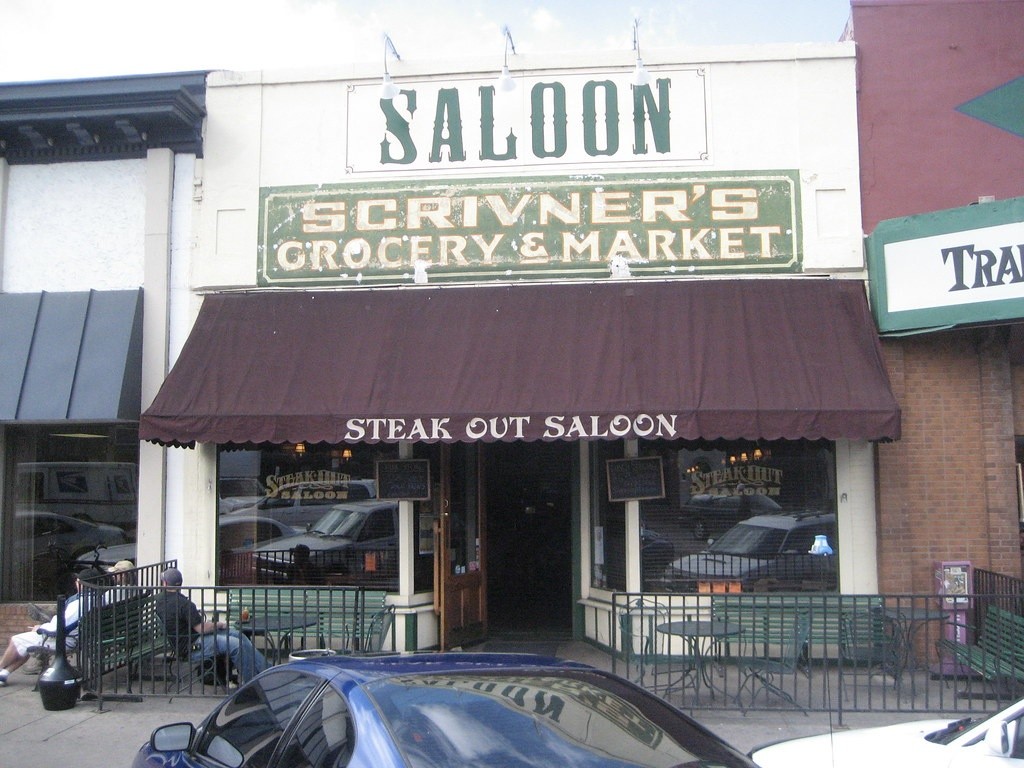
top-left (232, 615), bottom-right (320, 667)
top-left (865, 610), bottom-right (950, 696)
top-left (656, 620), bottom-right (741, 717)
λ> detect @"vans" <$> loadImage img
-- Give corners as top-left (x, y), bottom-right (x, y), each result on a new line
top-left (231, 479), bottom-right (377, 527)
top-left (13, 461), bottom-right (138, 524)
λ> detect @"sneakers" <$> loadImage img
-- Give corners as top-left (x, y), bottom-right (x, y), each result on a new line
top-left (0, 675), bottom-right (7, 686)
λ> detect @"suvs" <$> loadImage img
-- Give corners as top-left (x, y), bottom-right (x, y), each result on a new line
top-left (679, 491), bottom-right (782, 541)
top-left (662, 511), bottom-right (839, 591)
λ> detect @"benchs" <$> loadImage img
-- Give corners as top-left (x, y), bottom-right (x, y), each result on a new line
top-left (228, 590), bottom-right (386, 659)
top-left (711, 595), bottom-right (886, 678)
top-left (26, 587), bottom-right (152, 692)
top-left (930, 604), bottom-right (1024, 699)
top-left (79, 594), bottom-right (182, 703)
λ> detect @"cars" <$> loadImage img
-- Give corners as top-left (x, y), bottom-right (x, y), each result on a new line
top-left (73, 543), bottom-right (136, 572)
top-left (133, 650), bottom-right (767, 768)
top-left (748, 695), bottom-right (1024, 768)
top-left (641, 528), bottom-right (676, 573)
top-left (12, 511), bottom-right (125, 568)
top-left (219, 516), bottom-right (308, 578)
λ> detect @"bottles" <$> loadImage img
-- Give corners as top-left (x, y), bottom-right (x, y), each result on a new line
top-left (349, 573), bottom-right (365, 586)
top-left (420, 518), bottom-right (433, 550)
top-left (331, 565), bottom-right (347, 586)
top-left (365, 576), bottom-right (384, 591)
top-left (415, 550), bottom-right (434, 592)
top-left (351, 551), bottom-right (398, 574)
top-left (384, 575), bottom-right (399, 592)
top-left (242, 607), bottom-right (250, 622)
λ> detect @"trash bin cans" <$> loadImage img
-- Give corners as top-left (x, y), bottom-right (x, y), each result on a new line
top-left (288, 649), bottom-right (336, 713)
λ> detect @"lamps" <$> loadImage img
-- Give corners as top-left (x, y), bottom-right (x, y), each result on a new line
top-left (342, 450), bottom-right (352, 463)
top-left (495, 31), bottom-right (518, 91)
top-left (628, 20), bottom-right (651, 86)
top-left (379, 36), bottom-right (401, 100)
top-left (295, 444), bottom-right (305, 457)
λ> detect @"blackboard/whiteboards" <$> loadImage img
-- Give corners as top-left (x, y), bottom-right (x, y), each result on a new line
top-left (376, 458), bottom-right (430, 501)
top-left (606, 456), bottom-right (665, 502)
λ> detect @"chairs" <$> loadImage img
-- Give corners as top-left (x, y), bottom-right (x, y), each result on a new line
top-left (839, 609), bottom-right (908, 705)
top-left (345, 604), bottom-right (396, 654)
top-left (617, 598), bottom-right (702, 703)
top-left (732, 611), bottom-right (811, 717)
top-left (152, 610), bottom-right (229, 703)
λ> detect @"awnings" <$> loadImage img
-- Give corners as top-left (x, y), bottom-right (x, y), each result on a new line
top-left (0, 287), bottom-right (144, 423)
top-left (139, 279), bottom-right (902, 450)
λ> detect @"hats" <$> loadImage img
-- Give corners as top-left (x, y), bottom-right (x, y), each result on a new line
top-left (160, 568), bottom-right (183, 586)
top-left (75, 569), bottom-right (97, 585)
top-left (108, 561), bottom-right (135, 573)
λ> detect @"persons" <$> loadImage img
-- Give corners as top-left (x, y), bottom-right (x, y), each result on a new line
top-left (156, 569), bottom-right (274, 688)
top-left (0, 560), bottom-right (139, 687)
top-left (283, 545), bottom-right (327, 586)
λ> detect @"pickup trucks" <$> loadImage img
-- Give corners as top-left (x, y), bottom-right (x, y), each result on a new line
top-left (251, 500), bottom-right (433, 583)
